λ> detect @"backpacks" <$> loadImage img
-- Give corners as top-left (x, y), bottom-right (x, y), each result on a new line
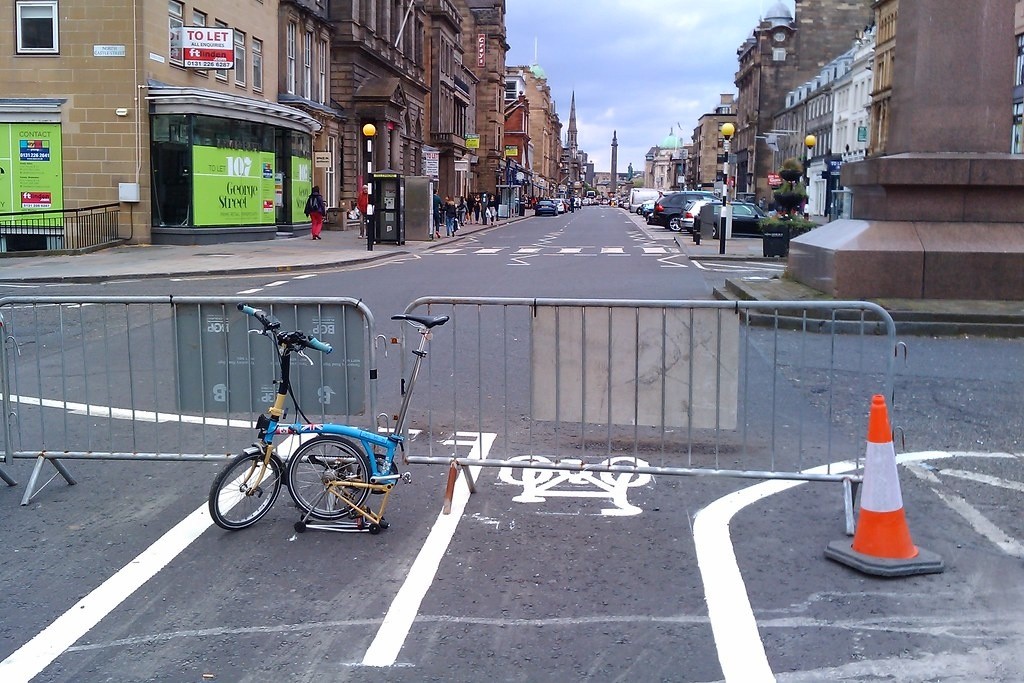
top-left (308, 194), bottom-right (323, 211)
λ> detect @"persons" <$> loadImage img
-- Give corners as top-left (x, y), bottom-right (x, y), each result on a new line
top-left (304, 185), bottom-right (326, 240)
top-left (441, 200), bottom-right (457, 238)
top-left (473, 194), bottom-right (482, 224)
top-left (520, 194), bottom-right (561, 210)
top-left (433, 188), bottom-right (443, 238)
top-left (486, 194), bottom-right (497, 225)
top-left (655, 192), bottom-right (665, 203)
top-left (356, 184), bottom-right (368, 239)
top-left (438, 193), bottom-right (470, 227)
top-left (467, 194), bottom-right (474, 225)
top-left (731, 197), bottom-right (805, 222)
top-left (578, 197), bottom-right (582, 210)
top-left (570, 196), bottom-right (575, 213)
top-left (576, 196), bottom-right (580, 210)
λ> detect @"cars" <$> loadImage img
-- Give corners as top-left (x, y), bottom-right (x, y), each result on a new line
top-left (535, 201), bottom-right (558, 216)
top-left (552, 187), bottom-right (671, 225)
top-left (694, 202), bottom-right (768, 239)
top-left (678, 199), bottom-right (722, 234)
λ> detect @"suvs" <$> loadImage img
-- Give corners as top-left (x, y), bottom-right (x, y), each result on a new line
top-left (655, 192), bottom-right (722, 232)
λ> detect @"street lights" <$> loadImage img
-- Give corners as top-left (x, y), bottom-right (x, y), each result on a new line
top-left (804, 134), bottom-right (816, 220)
top-left (363, 124), bottom-right (376, 250)
top-left (721, 122), bottom-right (734, 240)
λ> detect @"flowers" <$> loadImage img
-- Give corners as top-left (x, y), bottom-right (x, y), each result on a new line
top-left (757, 209), bottom-right (818, 230)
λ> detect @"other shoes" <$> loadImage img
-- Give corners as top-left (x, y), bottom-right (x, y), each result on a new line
top-left (314, 237), bottom-right (321, 239)
top-left (452, 231), bottom-right (454, 237)
top-left (436, 231), bottom-right (441, 238)
top-left (446, 236), bottom-right (450, 238)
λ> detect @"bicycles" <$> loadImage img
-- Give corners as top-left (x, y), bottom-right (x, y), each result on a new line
top-left (208, 304), bottom-right (450, 533)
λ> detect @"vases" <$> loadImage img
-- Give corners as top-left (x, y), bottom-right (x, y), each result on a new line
top-left (762, 227), bottom-right (811, 258)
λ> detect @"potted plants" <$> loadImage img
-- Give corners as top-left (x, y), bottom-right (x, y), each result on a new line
top-left (772, 182), bottom-right (806, 209)
top-left (773, 157), bottom-right (803, 180)
